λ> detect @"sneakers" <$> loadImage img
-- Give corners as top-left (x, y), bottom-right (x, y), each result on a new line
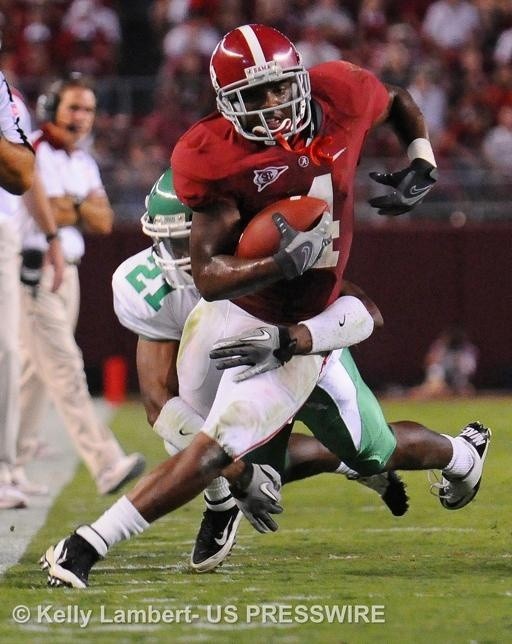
top-left (439, 422), bottom-right (491, 509)
top-left (95, 454), bottom-right (144, 496)
top-left (38, 522), bottom-right (109, 589)
top-left (348, 470), bottom-right (410, 517)
top-left (190, 497), bottom-right (242, 575)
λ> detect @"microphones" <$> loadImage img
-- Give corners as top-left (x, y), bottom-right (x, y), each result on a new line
top-left (54, 125), bottom-right (76, 132)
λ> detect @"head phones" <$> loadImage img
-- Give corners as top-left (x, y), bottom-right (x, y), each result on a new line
top-left (35, 80), bottom-right (66, 122)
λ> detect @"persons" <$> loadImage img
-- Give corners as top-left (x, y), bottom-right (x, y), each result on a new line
top-left (11, 74), bottom-right (145, 496)
top-left (0, 2), bottom-right (510, 208)
top-left (36, 24), bottom-right (439, 589)
top-left (0, 74), bottom-right (43, 510)
top-left (111, 159), bottom-right (491, 573)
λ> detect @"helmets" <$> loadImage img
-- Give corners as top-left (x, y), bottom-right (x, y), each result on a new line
top-left (141, 169), bottom-right (197, 291)
top-left (209, 24), bottom-right (313, 146)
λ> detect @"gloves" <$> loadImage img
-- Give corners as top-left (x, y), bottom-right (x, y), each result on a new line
top-left (271, 211), bottom-right (332, 277)
top-left (228, 462), bottom-right (283, 534)
top-left (368, 158), bottom-right (438, 217)
top-left (209, 326), bottom-right (282, 381)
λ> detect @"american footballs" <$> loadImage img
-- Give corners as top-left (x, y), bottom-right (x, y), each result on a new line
top-left (235, 195), bottom-right (330, 258)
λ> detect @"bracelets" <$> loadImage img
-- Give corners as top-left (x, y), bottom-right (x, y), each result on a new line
top-left (44, 233), bottom-right (60, 242)
